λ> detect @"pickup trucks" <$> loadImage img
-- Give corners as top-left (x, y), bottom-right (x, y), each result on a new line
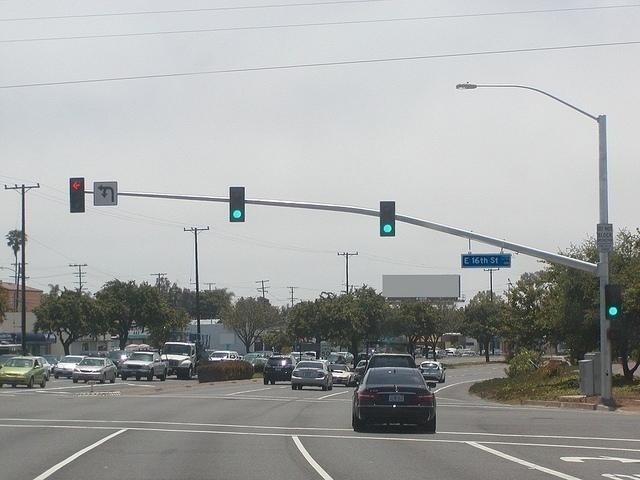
top-left (121, 351), bottom-right (169, 380)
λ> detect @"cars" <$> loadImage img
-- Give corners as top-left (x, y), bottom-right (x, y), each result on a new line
top-left (414, 344), bottom-right (485, 358)
top-left (73, 357), bottom-right (117, 384)
top-left (40, 343), bottom-right (158, 377)
top-left (0, 355), bottom-right (48, 388)
top-left (54, 354), bottom-right (88, 380)
top-left (352, 366), bottom-right (438, 431)
top-left (239, 349), bottom-right (368, 391)
top-left (421, 360), bottom-right (446, 383)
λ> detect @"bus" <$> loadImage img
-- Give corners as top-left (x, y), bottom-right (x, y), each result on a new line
top-left (0, 341), bottom-right (28, 367)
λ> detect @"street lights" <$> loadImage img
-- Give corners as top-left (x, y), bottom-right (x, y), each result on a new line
top-left (456, 83), bottom-right (616, 410)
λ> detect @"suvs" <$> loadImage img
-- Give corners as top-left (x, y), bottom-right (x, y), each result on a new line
top-left (359, 355), bottom-right (417, 375)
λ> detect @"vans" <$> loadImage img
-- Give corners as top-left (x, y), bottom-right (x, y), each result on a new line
top-left (208, 350), bottom-right (239, 362)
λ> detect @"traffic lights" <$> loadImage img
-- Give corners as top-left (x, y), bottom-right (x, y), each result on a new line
top-left (604, 282), bottom-right (622, 320)
top-left (69, 178), bottom-right (85, 213)
top-left (379, 201), bottom-right (396, 238)
top-left (229, 186), bottom-right (246, 223)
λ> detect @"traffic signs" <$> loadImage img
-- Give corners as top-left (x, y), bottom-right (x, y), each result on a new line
top-left (461, 255), bottom-right (512, 268)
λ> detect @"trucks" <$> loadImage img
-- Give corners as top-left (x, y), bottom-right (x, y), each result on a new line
top-left (155, 342), bottom-right (196, 378)
